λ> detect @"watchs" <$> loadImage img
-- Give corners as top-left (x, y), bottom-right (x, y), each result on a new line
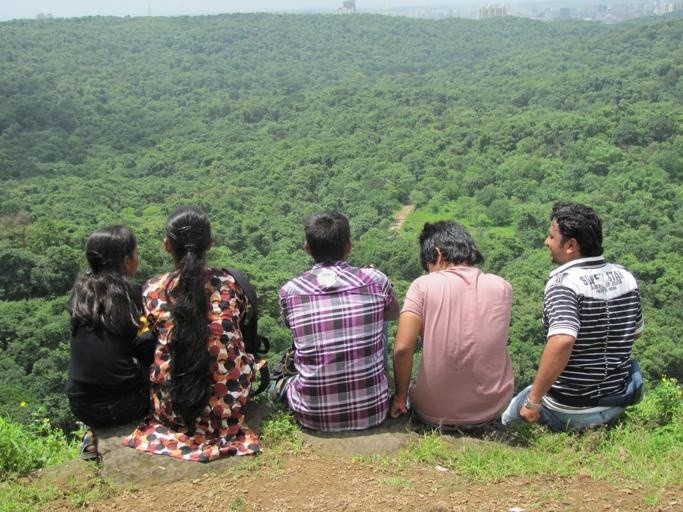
top-left (521, 397), bottom-right (543, 413)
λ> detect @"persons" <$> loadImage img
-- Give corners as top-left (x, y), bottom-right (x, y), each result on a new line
top-left (122, 207), bottom-right (269, 462)
top-left (388, 220), bottom-right (516, 434)
top-left (63, 224), bottom-right (159, 460)
top-left (498, 199), bottom-right (644, 432)
top-left (270, 210), bottom-right (400, 436)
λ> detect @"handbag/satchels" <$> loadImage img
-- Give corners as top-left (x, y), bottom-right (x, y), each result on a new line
top-left (597, 359), bottom-right (644, 408)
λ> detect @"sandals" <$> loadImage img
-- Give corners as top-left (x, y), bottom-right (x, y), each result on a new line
top-left (79, 428), bottom-right (98, 461)
